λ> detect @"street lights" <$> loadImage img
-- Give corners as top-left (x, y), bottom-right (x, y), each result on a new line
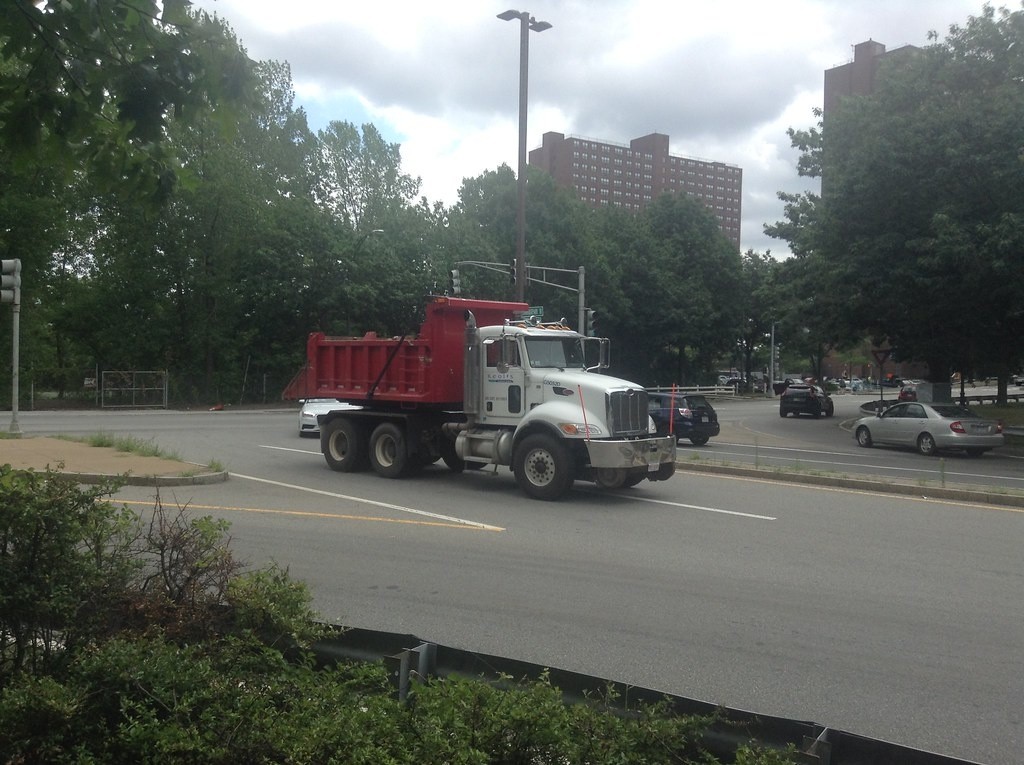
top-left (496, 10), bottom-right (553, 302)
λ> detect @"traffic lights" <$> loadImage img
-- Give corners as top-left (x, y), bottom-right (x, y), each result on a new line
top-left (448, 270), bottom-right (458, 294)
top-left (587, 311), bottom-right (599, 337)
top-left (0, 260), bottom-right (14, 303)
top-left (510, 259), bottom-right (517, 285)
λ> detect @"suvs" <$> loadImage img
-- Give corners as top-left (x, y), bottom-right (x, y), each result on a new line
top-left (647, 392), bottom-right (720, 446)
top-left (780, 385), bottom-right (834, 417)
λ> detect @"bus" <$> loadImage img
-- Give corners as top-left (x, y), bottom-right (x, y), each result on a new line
top-left (717, 370), bottom-right (747, 384)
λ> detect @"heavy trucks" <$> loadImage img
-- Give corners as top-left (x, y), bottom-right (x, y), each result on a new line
top-left (282, 297), bottom-right (676, 500)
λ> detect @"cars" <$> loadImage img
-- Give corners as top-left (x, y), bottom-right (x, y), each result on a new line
top-left (851, 402), bottom-right (1005, 456)
top-left (724, 378), bottom-right (914, 391)
top-left (898, 384), bottom-right (917, 402)
top-left (298, 398), bottom-right (363, 437)
